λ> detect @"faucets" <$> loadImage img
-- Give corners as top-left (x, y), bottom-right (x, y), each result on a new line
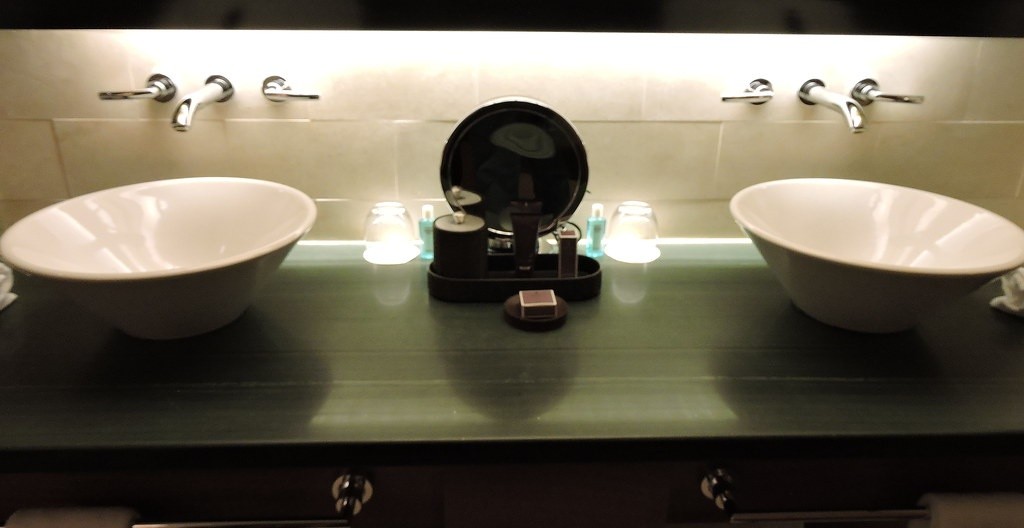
top-left (799, 81), bottom-right (866, 134)
top-left (170, 79), bottom-right (232, 133)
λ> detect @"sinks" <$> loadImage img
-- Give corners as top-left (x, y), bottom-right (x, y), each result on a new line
top-left (728, 176), bottom-right (1024, 330)
top-left (1, 176), bottom-right (318, 339)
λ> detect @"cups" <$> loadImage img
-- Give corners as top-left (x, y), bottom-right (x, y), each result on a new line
top-left (362, 201), bottom-right (420, 265)
top-left (601, 200), bottom-right (661, 263)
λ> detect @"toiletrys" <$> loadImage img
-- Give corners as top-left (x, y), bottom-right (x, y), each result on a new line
top-left (587, 203), bottom-right (606, 257)
top-left (418, 204), bottom-right (434, 260)
